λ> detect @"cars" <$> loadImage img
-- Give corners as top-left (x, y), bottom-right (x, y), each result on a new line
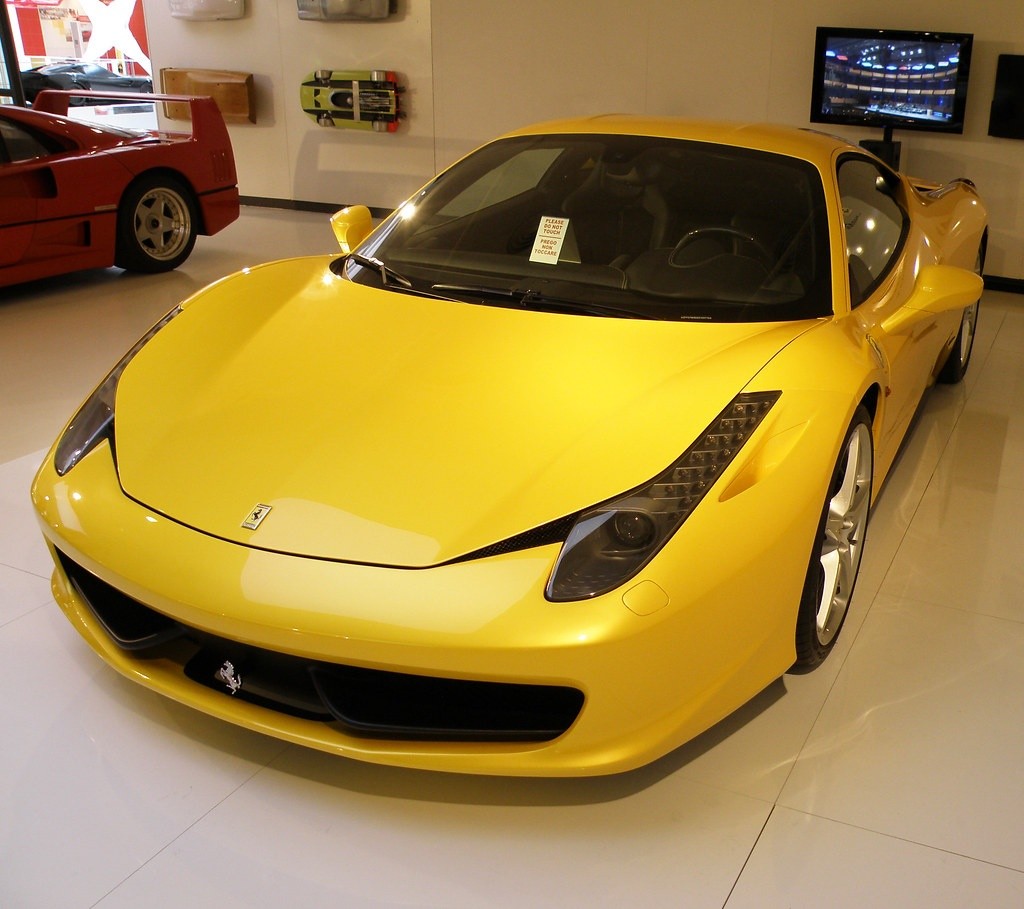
top-left (20, 62), bottom-right (155, 107)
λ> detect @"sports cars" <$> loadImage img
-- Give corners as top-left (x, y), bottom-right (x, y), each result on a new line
top-left (0, 90), bottom-right (239, 284)
top-left (30, 112), bottom-right (989, 778)
top-left (300, 70), bottom-right (406, 134)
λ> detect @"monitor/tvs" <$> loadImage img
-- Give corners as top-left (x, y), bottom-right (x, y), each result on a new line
top-left (810, 26), bottom-right (974, 134)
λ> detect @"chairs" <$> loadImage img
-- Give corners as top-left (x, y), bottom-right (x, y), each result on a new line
top-left (731, 170), bottom-right (810, 281)
top-left (565, 150), bottom-right (670, 267)
